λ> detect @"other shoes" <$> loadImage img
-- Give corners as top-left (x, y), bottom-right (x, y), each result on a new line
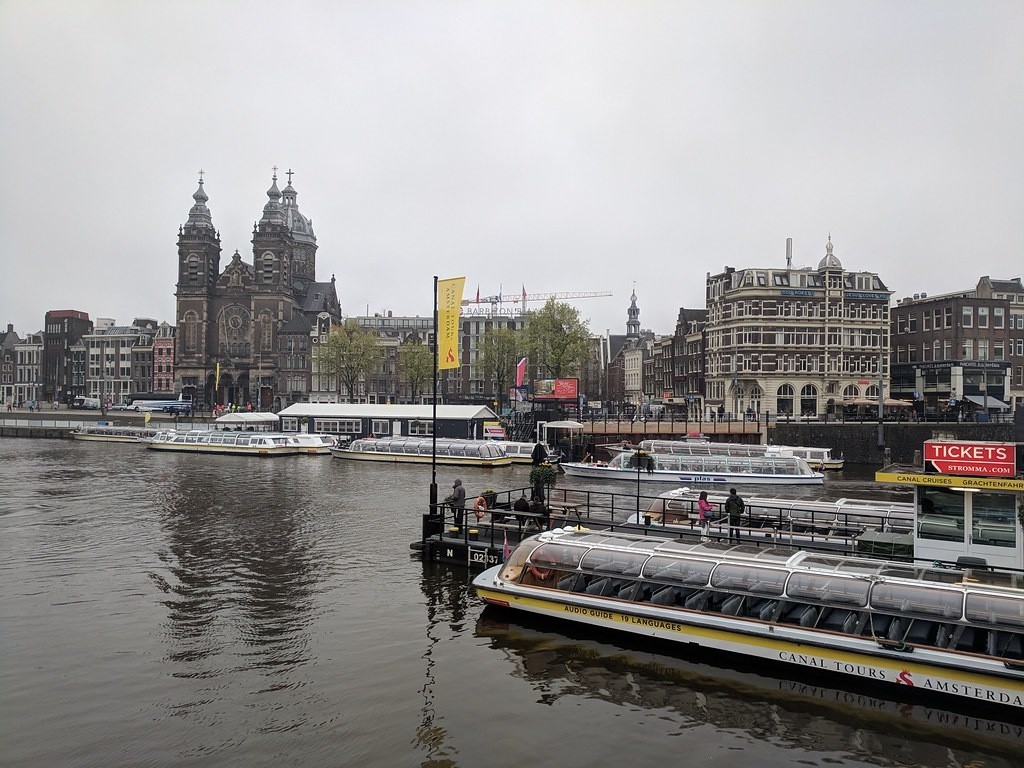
top-left (737, 541), bottom-right (743, 545)
top-left (726, 541), bottom-right (732, 544)
top-left (700, 537), bottom-right (711, 542)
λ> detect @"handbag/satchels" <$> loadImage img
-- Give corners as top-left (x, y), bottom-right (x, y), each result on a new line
top-left (739, 506), bottom-right (743, 513)
top-left (703, 510), bottom-right (714, 518)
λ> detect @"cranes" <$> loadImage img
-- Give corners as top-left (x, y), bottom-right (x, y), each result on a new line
top-left (468, 289), bottom-right (612, 321)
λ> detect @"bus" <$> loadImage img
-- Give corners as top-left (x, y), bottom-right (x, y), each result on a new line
top-left (124, 392), bottom-right (194, 413)
top-left (73, 397), bottom-right (101, 410)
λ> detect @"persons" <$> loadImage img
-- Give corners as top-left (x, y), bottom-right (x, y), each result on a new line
top-left (247, 401), bottom-right (252, 411)
top-left (449, 479), bottom-right (466, 534)
top-left (697, 491), bottom-right (718, 542)
top-left (724, 488), bottom-right (746, 545)
top-left (184, 404), bottom-right (191, 417)
top-left (514, 494), bottom-right (550, 530)
top-left (745, 405), bottom-right (753, 422)
top-left (717, 404), bottom-right (725, 423)
top-left (28, 401), bottom-right (35, 413)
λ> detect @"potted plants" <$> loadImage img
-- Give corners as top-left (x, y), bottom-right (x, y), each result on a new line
top-left (479, 489), bottom-right (497, 508)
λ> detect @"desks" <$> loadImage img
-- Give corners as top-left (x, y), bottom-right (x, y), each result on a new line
top-left (484, 509), bottom-right (543, 541)
top-left (544, 501), bottom-right (584, 529)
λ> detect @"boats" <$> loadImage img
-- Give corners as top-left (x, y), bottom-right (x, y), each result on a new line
top-left (557, 453), bottom-right (825, 486)
top-left (67, 427), bottom-right (561, 468)
top-left (468, 524), bottom-right (1023, 753)
top-left (595, 437), bottom-right (845, 472)
top-left (625, 484), bottom-right (917, 552)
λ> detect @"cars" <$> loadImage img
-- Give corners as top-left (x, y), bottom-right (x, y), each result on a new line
top-left (112, 403), bottom-right (127, 411)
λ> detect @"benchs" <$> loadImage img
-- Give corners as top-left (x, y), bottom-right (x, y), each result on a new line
top-left (550, 507), bottom-right (589, 514)
top-left (550, 514), bottom-right (571, 519)
top-left (478, 522), bottom-right (547, 532)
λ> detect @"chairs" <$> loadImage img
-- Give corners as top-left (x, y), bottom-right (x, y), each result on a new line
top-left (556, 562), bottom-right (1024, 661)
top-left (372, 447), bottom-right (478, 456)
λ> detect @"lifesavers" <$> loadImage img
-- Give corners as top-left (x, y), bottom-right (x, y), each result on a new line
top-left (528, 550), bottom-right (556, 579)
top-left (472, 496), bottom-right (486, 518)
